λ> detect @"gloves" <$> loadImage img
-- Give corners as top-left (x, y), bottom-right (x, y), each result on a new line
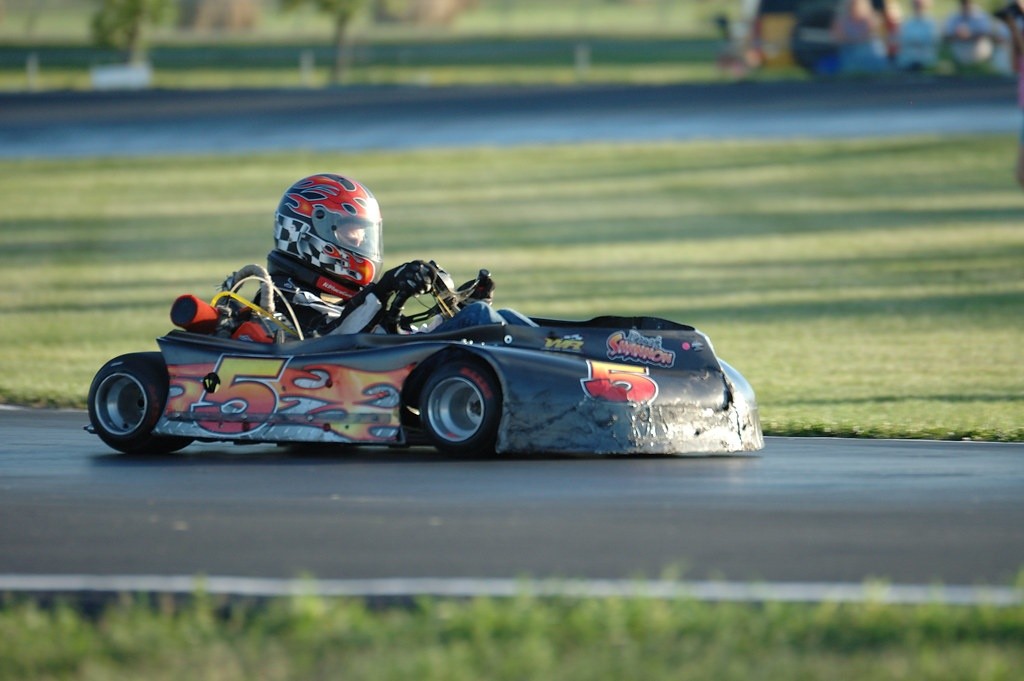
top-left (376, 260), bottom-right (434, 298)
top-left (457, 275), bottom-right (496, 309)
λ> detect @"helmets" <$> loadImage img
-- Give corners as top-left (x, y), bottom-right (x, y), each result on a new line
top-left (273, 174), bottom-right (385, 293)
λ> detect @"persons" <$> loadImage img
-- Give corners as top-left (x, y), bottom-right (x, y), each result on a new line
top-left (831, 0), bottom-right (888, 70)
top-left (993, 0), bottom-right (1024, 72)
top-left (253, 173), bottom-right (538, 341)
top-left (892, 0), bottom-right (941, 70)
top-left (945, 0), bottom-right (1009, 74)
